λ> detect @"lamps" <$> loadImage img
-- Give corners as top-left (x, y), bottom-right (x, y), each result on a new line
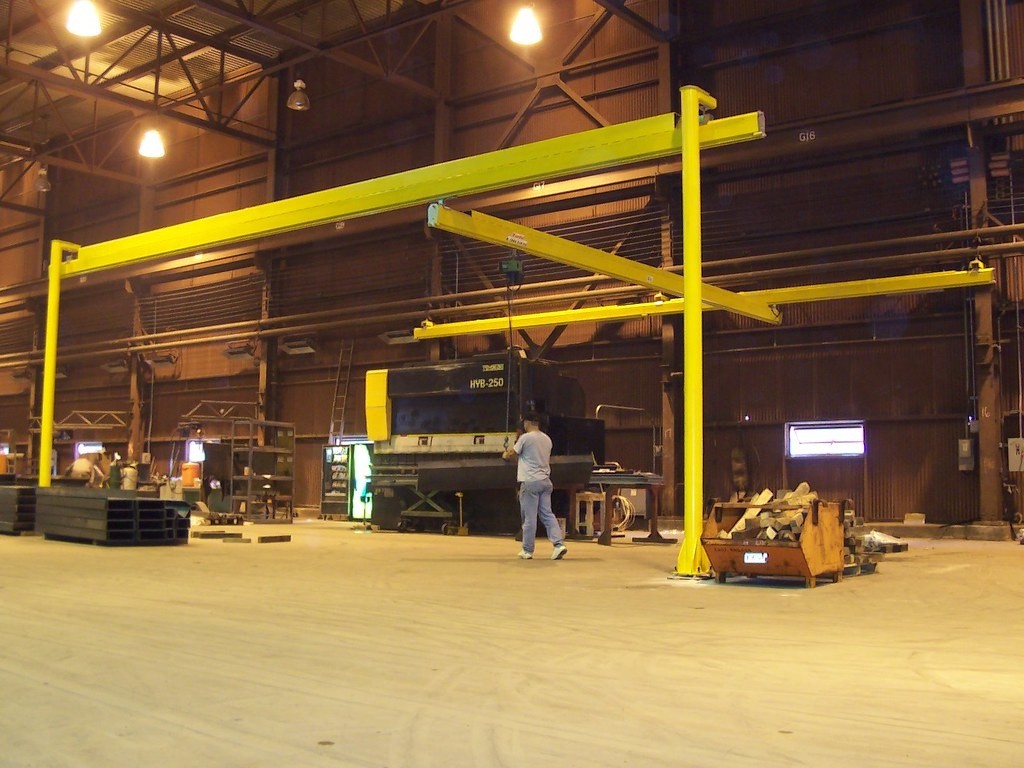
top-left (286, 8), bottom-right (311, 112)
top-left (137, 112), bottom-right (165, 159)
top-left (377, 322), bottom-right (421, 346)
top-left (143, 349), bottom-right (179, 367)
top-left (222, 340), bottom-right (255, 360)
top-left (66, 0), bottom-right (103, 38)
top-left (276, 334), bottom-right (318, 354)
top-left (509, 0), bottom-right (543, 47)
top-left (33, 113), bottom-right (53, 194)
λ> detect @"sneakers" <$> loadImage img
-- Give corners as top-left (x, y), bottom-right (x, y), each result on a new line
top-left (517, 551), bottom-right (532, 559)
top-left (551, 546), bottom-right (567, 560)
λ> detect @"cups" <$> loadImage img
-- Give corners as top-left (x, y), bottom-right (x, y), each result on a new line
top-left (193, 478), bottom-right (200, 487)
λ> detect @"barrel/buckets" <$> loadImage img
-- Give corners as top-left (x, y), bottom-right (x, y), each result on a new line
top-left (110, 465), bottom-right (124, 489)
top-left (182, 462), bottom-right (200, 486)
top-left (120, 468), bottom-right (138, 490)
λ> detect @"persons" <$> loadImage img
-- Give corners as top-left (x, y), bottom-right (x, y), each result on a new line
top-left (514, 411), bottom-right (567, 561)
top-left (66, 455), bottom-right (94, 485)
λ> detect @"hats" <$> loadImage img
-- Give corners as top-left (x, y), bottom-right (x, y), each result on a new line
top-left (521, 411), bottom-right (539, 423)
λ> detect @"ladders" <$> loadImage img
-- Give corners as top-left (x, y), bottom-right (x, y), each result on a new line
top-left (328, 338), bottom-right (354, 446)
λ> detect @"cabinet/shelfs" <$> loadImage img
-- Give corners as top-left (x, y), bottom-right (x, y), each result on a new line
top-left (320, 444), bottom-right (354, 522)
top-left (228, 418), bottom-right (296, 524)
